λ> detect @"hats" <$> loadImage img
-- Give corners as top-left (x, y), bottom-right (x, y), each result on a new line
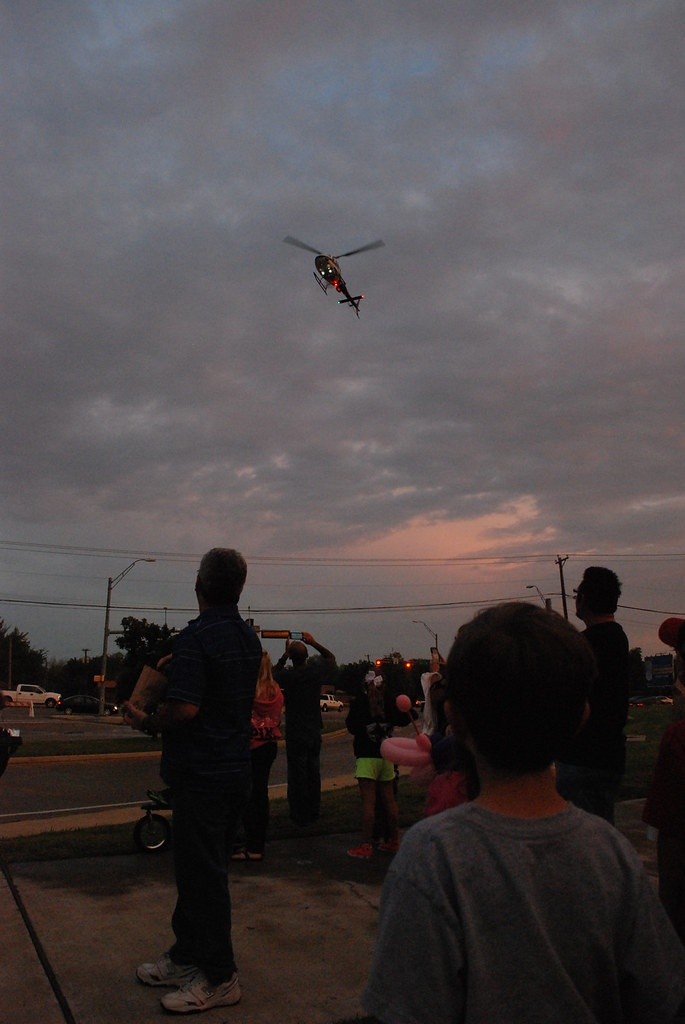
top-left (658, 617), bottom-right (685, 658)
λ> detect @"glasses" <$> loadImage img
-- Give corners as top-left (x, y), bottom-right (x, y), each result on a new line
top-left (571, 588), bottom-right (586, 599)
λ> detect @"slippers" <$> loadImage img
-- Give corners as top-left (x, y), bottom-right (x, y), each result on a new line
top-left (230, 846), bottom-right (265, 862)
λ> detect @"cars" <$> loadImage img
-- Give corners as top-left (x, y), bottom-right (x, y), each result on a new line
top-left (628, 696), bottom-right (647, 707)
top-left (655, 695), bottom-right (673, 705)
top-left (55, 695), bottom-right (118, 717)
top-left (632, 697), bottom-right (665, 707)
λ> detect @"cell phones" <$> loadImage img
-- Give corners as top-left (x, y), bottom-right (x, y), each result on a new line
top-left (430, 647), bottom-right (440, 662)
top-left (290, 632), bottom-right (303, 640)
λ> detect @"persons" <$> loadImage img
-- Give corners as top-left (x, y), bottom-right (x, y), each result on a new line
top-left (642, 618), bottom-right (685, 946)
top-left (124, 548), bottom-right (262, 1014)
top-left (359, 602), bottom-right (685, 1024)
top-left (271, 633), bottom-right (339, 824)
top-left (342, 670), bottom-right (400, 861)
top-left (556, 567), bottom-right (630, 827)
top-left (231, 655), bottom-right (284, 861)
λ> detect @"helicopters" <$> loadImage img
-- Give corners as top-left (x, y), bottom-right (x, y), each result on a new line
top-left (283, 236), bottom-right (386, 319)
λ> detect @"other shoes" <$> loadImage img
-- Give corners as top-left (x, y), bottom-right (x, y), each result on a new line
top-left (347, 842), bottom-right (373, 860)
top-left (375, 839), bottom-right (402, 852)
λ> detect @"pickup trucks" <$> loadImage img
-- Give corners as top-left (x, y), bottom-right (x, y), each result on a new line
top-left (0, 684), bottom-right (61, 708)
top-left (320, 694), bottom-right (343, 712)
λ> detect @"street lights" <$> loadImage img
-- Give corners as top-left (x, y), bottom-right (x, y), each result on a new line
top-left (99, 558), bottom-right (156, 713)
top-left (526, 585), bottom-right (552, 610)
top-left (413, 620), bottom-right (438, 648)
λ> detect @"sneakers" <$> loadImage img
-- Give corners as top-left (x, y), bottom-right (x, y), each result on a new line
top-left (160, 966), bottom-right (244, 1014)
top-left (134, 955), bottom-right (202, 990)
